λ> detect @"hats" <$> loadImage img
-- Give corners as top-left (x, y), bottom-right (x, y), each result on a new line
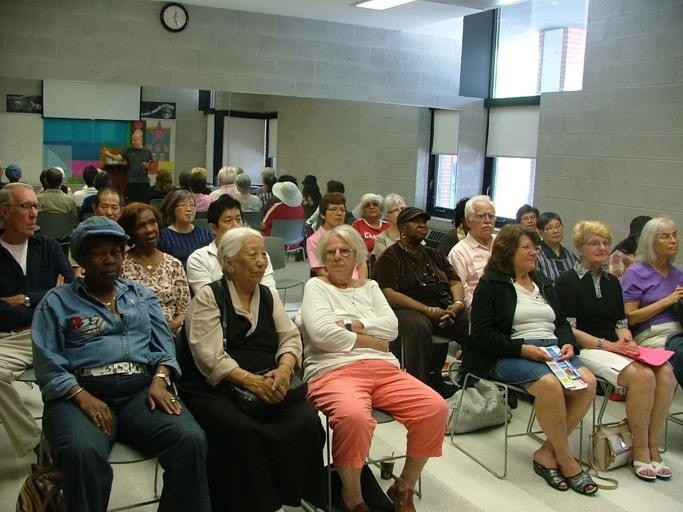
top-left (397, 207), bottom-right (430, 227)
top-left (71, 216), bottom-right (128, 259)
top-left (17, 463), bottom-right (68, 511)
top-left (351, 193), bottom-right (384, 220)
top-left (272, 181), bottom-right (302, 208)
top-left (628, 216), bottom-right (654, 238)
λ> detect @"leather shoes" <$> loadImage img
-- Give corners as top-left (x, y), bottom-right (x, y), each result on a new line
top-left (387, 480), bottom-right (415, 512)
top-left (340, 491), bottom-right (372, 512)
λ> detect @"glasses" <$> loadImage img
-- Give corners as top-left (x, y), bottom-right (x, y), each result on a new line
top-left (174, 203), bottom-right (195, 209)
top-left (321, 248), bottom-right (354, 258)
top-left (2, 202), bottom-right (41, 212)
top-left (655, 233), bottom-right (676, 241)
top-left (542, 225), bottom-right (564, 232)
top-left (388, 206), bottom-right (405, 213)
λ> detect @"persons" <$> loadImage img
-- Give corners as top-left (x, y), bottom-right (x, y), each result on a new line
top-left (102, 130), bottom-right (153, 204)
top-left (1, 161), bottom-right (450, 512)
top-left (436, 196), bottom-right (682, 495)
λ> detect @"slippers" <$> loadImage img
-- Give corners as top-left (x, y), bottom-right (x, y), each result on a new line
top-left (633, 460), bottom-right (656, 481)
top-left (559, 460), bottom-right (598, 496)
top-left (533, 458), bottom-right (569, 490)
top-left (651, 459), bottom-right (672, 480)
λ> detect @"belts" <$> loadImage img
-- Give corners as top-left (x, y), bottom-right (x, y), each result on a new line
top-left (77, 362), bottom-right (149, 375)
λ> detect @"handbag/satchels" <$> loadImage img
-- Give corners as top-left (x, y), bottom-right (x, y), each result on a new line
top-left (443, 378), bottom-right (512, 435)
top-left (234, 366), bottom-right (306, 409)
top-left (590, 420), bottom-right (635, 469)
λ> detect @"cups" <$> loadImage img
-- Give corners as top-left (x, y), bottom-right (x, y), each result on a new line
top-left (380, 455), bottom-right (396, 479)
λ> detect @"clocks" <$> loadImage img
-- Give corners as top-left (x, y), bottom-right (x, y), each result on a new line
top-left (160, 2), bottom-right (189, 33)
